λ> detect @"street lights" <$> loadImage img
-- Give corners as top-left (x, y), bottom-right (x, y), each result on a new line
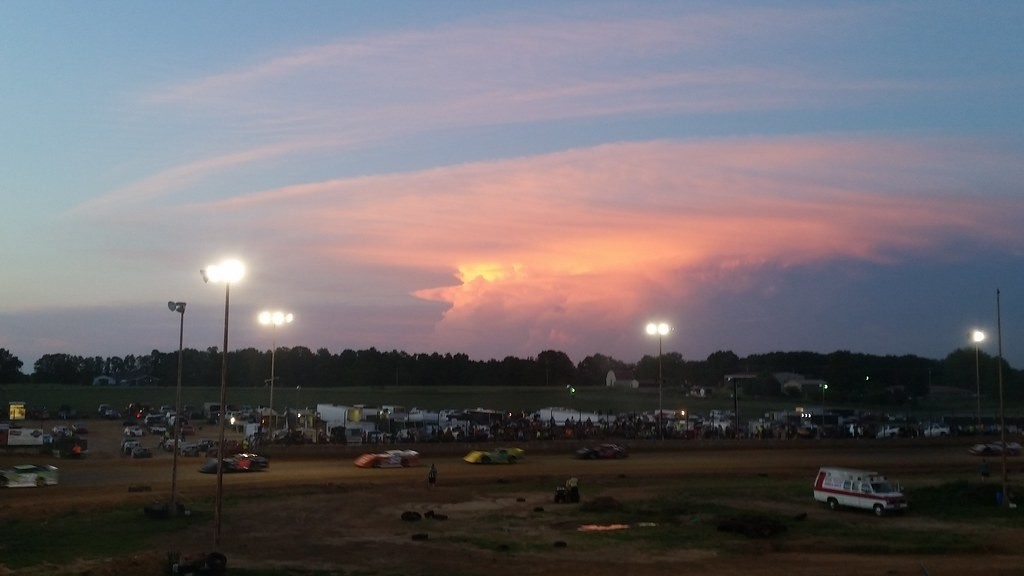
top-left (254, 306), bottom-right (295, 434)
top-left (645, 316), bottom-right (670, 434)
top-left (970, 328), bottom-right (988, 423)
top-left (168, 302), bottom-right (186, 512)
top-left (819, 383), bottom-right (828, 431)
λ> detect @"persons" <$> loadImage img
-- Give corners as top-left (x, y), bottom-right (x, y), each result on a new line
top-left (428, 463), bottom-right (437, 484)
top-left (430, 412), bottom-right (1024, 443)
top-left (565, 472), bottom-right (579, 503)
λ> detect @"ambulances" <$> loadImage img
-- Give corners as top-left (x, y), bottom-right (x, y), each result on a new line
top-left (814, 466), bottom-right (908, 517)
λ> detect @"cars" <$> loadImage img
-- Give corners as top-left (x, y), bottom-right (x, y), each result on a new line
top-left (122, 420), bottom-right (143, 437)
top-left (876, 420), bottom-right (951, 438)
top-left (165, 439), bottom-right (245, 457)
top-left (576, 445), bottom-right (630, 459)
top-left (145, 405), bottom-right (196, 435)
top-left (43, 422), bottom-right (88, 459)
top-left (463, 449), bottom-right (526, 465)
top-left (121, 439), bottom-right (153, 458)
top-left (355, 449), bottom-right (421, 468)
top-left (970, 442), bottom-right (1021, 457)
top-left (232, 451), bottom-right (270, 474)
top-left (0, 465), bottom-right (58, 488)
